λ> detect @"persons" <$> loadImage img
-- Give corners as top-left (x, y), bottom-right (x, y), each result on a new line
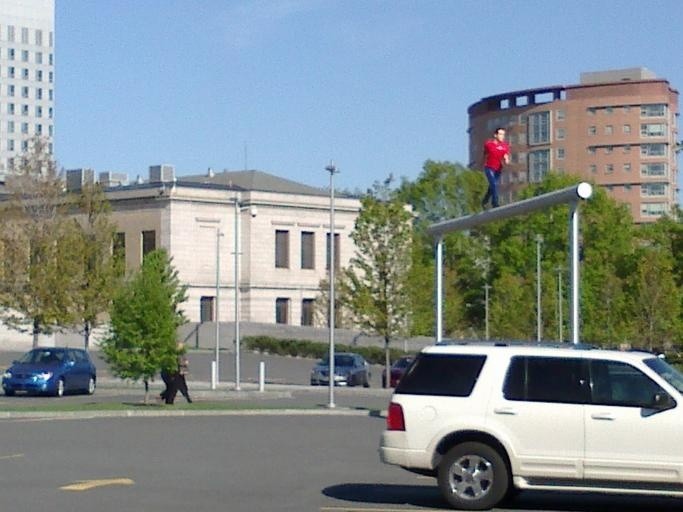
top-left (158, 367), bottom-right (171, 400)
top-left (481, 126), bottom-right (511, 209)
top-left (166, 342), bottom-right (191, 406)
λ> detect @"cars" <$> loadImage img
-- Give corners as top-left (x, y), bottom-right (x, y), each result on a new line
top-left (2, 347), bottom-right (96, 397)
top-left (311, 352), bottom-right (371, 387)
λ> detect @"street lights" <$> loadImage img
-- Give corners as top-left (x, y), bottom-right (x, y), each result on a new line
top-left (195, 215), bottom-right (224, 384)
top-left (481, 234), bottom-right (564, 343)
top-left (325, 159), bottom-right (340, 408)
